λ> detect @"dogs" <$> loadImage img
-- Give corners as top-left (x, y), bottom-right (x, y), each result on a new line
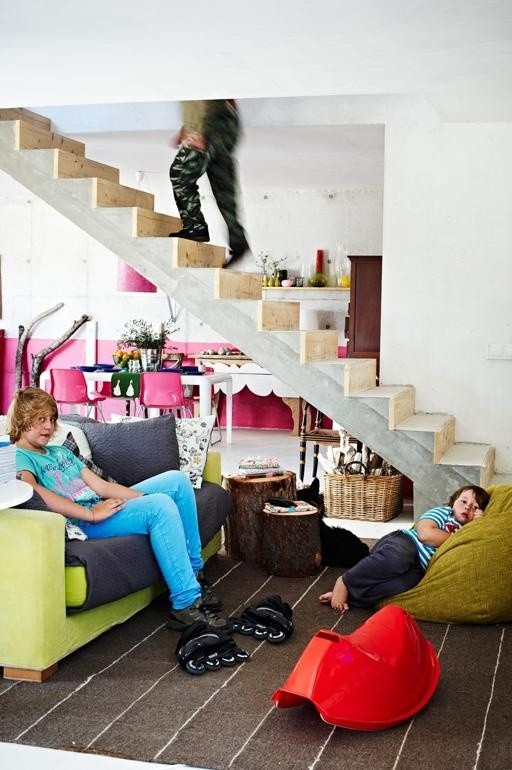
top-left (296, 477), bottom-right (369, 569)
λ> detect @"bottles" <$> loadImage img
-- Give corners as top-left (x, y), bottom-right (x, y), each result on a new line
top-left (309, 249), bottom-right (327, 287)
top-left (326, 258), bottom-right (351, 287)
top-left (262, 272), bottom-right (280, 287)
top-left (301, 263), bottom-right (307, 287)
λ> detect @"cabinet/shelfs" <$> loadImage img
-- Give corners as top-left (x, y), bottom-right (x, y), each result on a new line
top-left (344, 254), bottom-right (382, 389)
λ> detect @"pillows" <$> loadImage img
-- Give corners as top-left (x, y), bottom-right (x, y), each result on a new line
top-left (81, 407), bottom-right (179, 488)
top-left (106, 413), bottom-right (218, 491)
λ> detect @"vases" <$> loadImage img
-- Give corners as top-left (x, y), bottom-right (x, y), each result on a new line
top-left (332, 241), bottom-right (351, 288)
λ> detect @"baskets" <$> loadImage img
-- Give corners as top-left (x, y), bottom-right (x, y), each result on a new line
top-left (323, 461), bottom-right (403, 522)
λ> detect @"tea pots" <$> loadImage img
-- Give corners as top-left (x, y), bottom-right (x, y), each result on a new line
top-left (281, 279), bottom-right (294, 287)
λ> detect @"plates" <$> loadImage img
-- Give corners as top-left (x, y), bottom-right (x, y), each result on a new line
top-left (69, 363), bottom-right (122, 373)
top-left (158, 365), bottom-right (203, 375)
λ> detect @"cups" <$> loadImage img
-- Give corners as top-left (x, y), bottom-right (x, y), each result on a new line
top-left (288, 277), bottom-right (295, 286)
top-left (197, 364), bottom-right (206, 374)
top-left (278, 270), bottom-right (288, 281)
top-left (294, 277), bottom-right (304, 287)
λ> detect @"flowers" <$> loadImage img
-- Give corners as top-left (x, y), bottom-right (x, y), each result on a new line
top-left (120, 317), bottom-right (182, 349)
top-left (111, 345), bottom-right (141, 371)
top-left (256, 252), bottom-right (288, 287)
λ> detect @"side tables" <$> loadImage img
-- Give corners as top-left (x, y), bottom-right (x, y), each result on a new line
top-left (221, 470), bottom-right (299, 566)
top-left (259, 500), bottom-right (324, 581)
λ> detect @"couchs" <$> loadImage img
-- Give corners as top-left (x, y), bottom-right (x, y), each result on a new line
top-left (0, 411), bottom-right (236, 685)
top-left (368, 483), bottom-right (510, 630)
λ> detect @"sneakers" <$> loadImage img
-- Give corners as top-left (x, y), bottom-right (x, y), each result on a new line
top-left (166, 571), bottom-right (235, 633)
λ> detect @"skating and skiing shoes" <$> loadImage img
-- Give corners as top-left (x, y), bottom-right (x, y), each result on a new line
top-left (233, 595), bottom-right (294, 643)
top-left (176, 620), bottom-right (250, 674)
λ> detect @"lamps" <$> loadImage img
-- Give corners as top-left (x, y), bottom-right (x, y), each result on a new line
top-left (115, 255), bottom-right (177, 322)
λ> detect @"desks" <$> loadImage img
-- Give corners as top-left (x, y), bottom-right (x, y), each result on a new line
top-left (186, 355), bottom-right (316, 436)
top-left (39, 369), bottom-right (233, 446)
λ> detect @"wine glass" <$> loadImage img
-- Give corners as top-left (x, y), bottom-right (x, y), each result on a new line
top-left (128, 359), bottom-right (141, 374)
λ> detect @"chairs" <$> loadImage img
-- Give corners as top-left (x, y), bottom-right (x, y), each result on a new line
top-left (139, 371), bottom-right (196, 425)
top-left (184, 367), bottom-right (223, 446)
top-left (297, 397), bottom-right (368, 484)
top-left (49, 368), bottom-right (107, 424)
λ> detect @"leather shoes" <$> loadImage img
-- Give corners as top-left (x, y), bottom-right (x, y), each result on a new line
top-left (222, 239), bottom-right (248, 268)
top-left (169, 228), bottom-right (210, 242)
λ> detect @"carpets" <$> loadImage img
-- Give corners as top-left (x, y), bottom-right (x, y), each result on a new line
top-left (0, 536), bottom-right (509, 769)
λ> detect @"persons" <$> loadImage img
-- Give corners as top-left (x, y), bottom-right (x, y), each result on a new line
top-left (315, 484), bottom-right (491, 612)
top-left (165, 98), bottom-right (253, 273)
top-left (8, 385), bottom-right (224, 634)
